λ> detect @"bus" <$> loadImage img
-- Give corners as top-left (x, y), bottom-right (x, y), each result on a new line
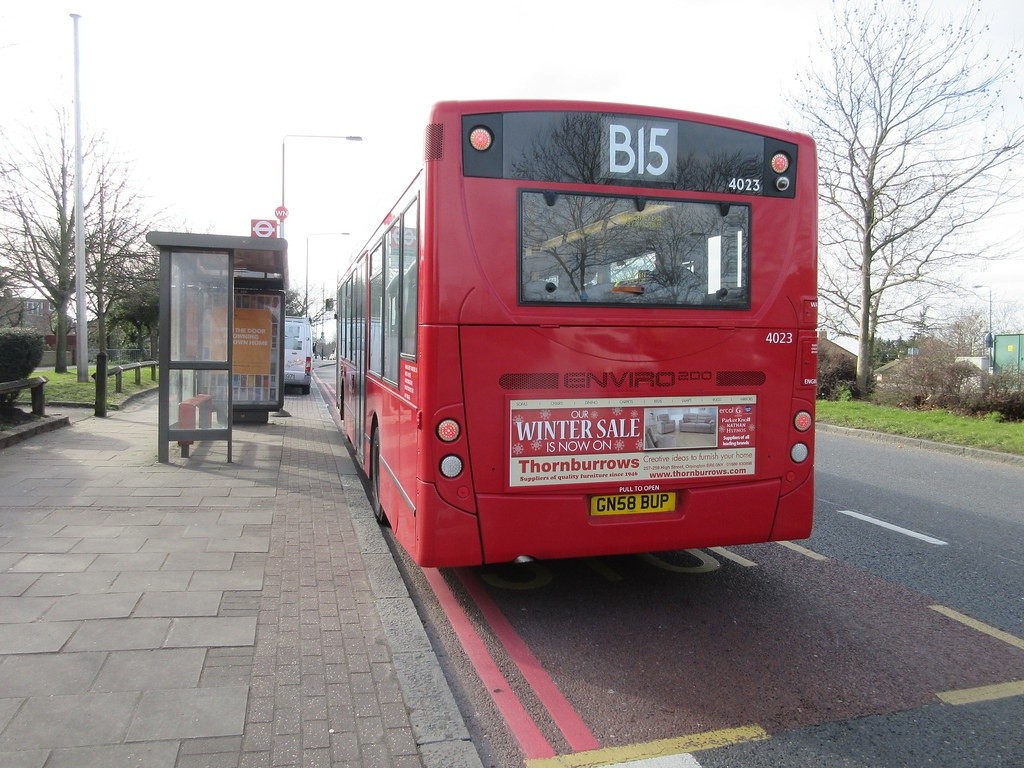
top-left (325, 99), bottom-right (819, 568)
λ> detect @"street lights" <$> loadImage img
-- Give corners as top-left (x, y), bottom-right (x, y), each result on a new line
top-left (67, 13), bottom-right (89, 383)
top-left (973, 285), bottom-right (992, 366)
top-left (280, 136), bottom-right (364, 240)
top-left (305, 231), bottom-right (351, 318)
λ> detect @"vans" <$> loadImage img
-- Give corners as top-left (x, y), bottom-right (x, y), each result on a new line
top-left (284, 315), bottom-right (316, 395)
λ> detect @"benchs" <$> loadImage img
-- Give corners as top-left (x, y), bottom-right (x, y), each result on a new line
top-left (178, 394), bottom-right (212, 457)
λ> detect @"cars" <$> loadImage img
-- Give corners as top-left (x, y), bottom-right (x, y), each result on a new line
top-left (329, 354), bottom-right (335, 360)
